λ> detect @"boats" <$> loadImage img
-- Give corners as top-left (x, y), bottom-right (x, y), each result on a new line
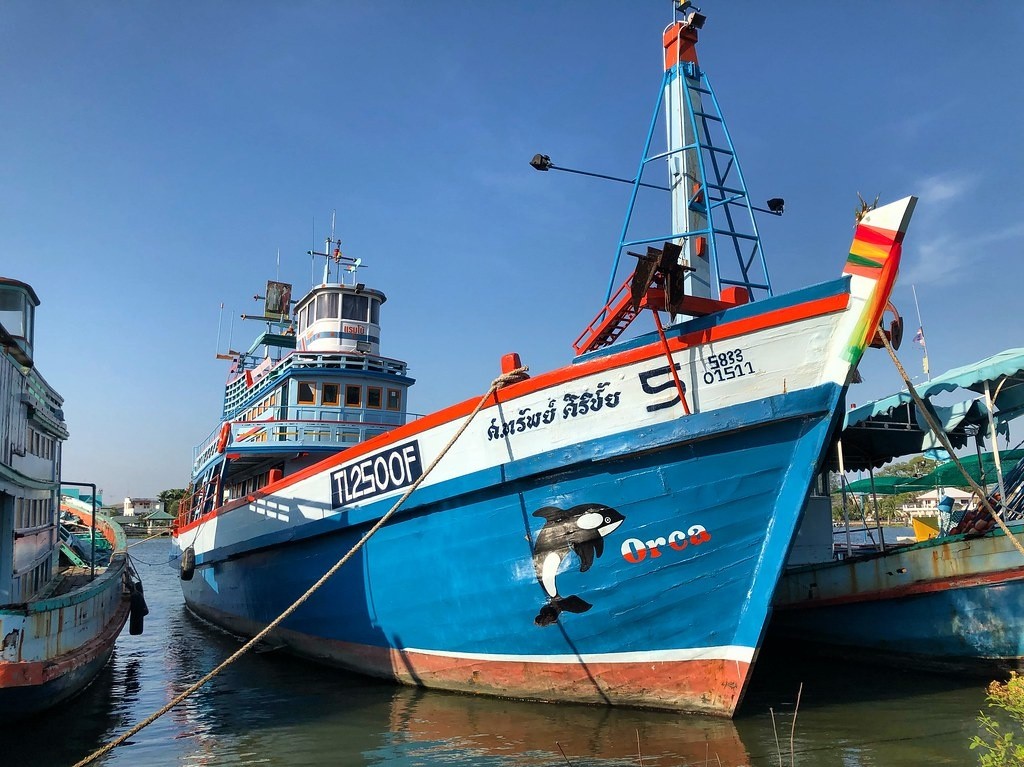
top-left (167, 0), bottom-right (918, 721)
top-left (0, 275), bottom-right (150, 741)
top-left (760, 344), bottom-right (1024, 676)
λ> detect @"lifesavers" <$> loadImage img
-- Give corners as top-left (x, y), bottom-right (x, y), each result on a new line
top-left (180, 547), bottom-right (195, 581)
top-left (218, 422), bottom-right (231, 453)
